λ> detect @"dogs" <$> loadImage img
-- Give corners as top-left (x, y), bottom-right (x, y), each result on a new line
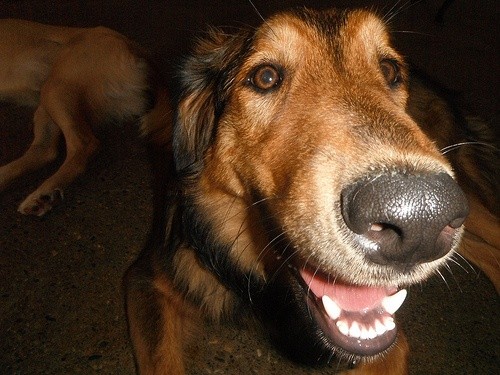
top-left (0, 16), bottom-right (181, 219)
top-left (123, 1), bottom-right (500, 375)
